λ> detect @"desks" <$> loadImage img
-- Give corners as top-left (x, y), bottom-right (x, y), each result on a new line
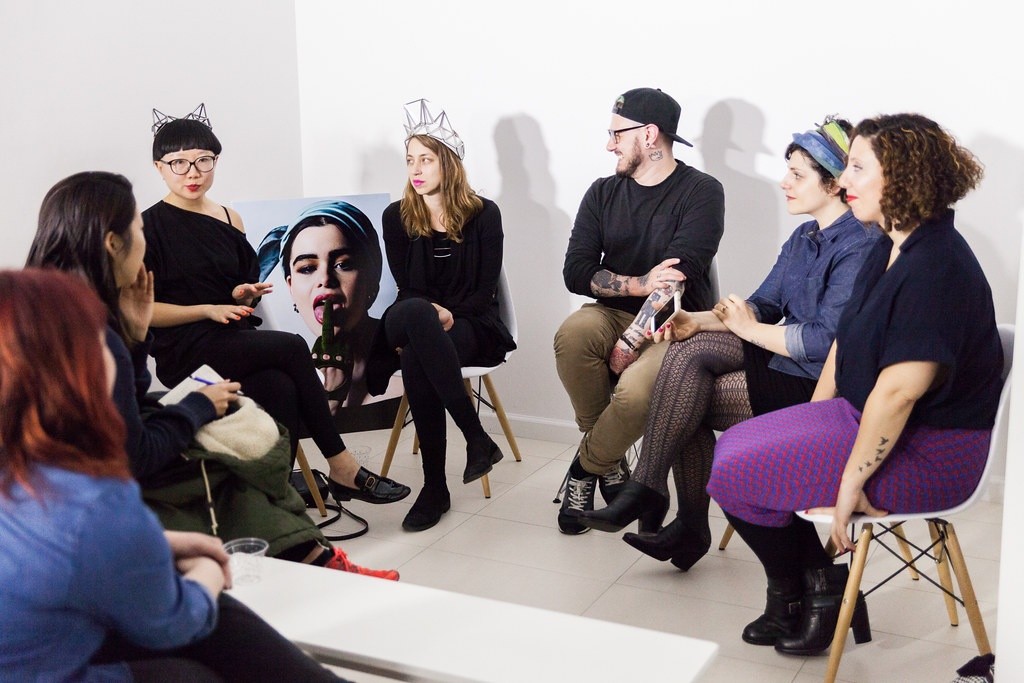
top-left (176, 548), bottom-right (721, 683)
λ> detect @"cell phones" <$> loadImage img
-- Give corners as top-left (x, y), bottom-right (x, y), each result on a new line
top-left (650, 291), bottom-right (681, 334)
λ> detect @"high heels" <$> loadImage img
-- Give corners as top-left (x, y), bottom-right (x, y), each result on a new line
top-left (622, 510), bottom-right (712, 572)
top-left (742, 561), bottom-right (872, 655)
top-left (579, 481), bottom-right (669, 534)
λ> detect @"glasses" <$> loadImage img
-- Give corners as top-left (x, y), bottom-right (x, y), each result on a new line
top-left (608, 124), bottom-right (648, 144)
top-left (160, 154), bottom-right (215, 175)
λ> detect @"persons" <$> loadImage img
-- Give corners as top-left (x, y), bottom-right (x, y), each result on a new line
top-left (141, 117), bottom-right (410, 505)
top-left (557, 89), bottom-right (724, 533)
top-left (383, 134), bottom-right (519, 533)
top-left (250, 200), bottom-right (405, 413)
top-left (0, 268), bottom-right (354, 683)
top-left (564, 119), bottom-right (883, 572)
top-left (706, 111), bottom-right (1004, 654)
top-left (20, 171), bottom-right (399, 583)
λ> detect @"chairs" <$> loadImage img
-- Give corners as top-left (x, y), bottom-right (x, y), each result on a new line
top-left (165, 242), bottom-right (1016, 683)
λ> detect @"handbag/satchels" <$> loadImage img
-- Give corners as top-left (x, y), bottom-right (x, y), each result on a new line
top-left (288, 469), bottom-right (329, 504)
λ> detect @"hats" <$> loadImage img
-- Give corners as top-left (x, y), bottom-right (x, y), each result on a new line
top-left (611, 89), bottom-right (693, 147)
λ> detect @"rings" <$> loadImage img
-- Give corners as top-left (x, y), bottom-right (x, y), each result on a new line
top-left (721, 305), bottom-right (728, 312)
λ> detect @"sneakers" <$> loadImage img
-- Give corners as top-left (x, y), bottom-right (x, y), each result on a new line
top-left (323, 546), bottom-right (399, 582)
top-left (557, 455), bottom-right (632, 535)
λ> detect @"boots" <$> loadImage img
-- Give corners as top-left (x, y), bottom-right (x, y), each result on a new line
top-left (449, 396), bottom-right (504, 484)
top-left (402, 439), bottom-right (450, 532)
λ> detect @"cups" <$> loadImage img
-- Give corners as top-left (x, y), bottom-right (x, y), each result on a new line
top-left (223, 537), bottom-right (269, 600)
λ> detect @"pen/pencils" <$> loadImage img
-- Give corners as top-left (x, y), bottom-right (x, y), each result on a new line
top-left (190, 375), bottom-right (244, 395)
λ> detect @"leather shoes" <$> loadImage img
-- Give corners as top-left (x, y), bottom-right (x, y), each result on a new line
top-left (328, 465), bottom-right (411, 505)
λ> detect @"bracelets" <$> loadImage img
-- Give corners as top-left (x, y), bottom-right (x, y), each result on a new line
top-left (619, 335), bottom-right (639, 351)
